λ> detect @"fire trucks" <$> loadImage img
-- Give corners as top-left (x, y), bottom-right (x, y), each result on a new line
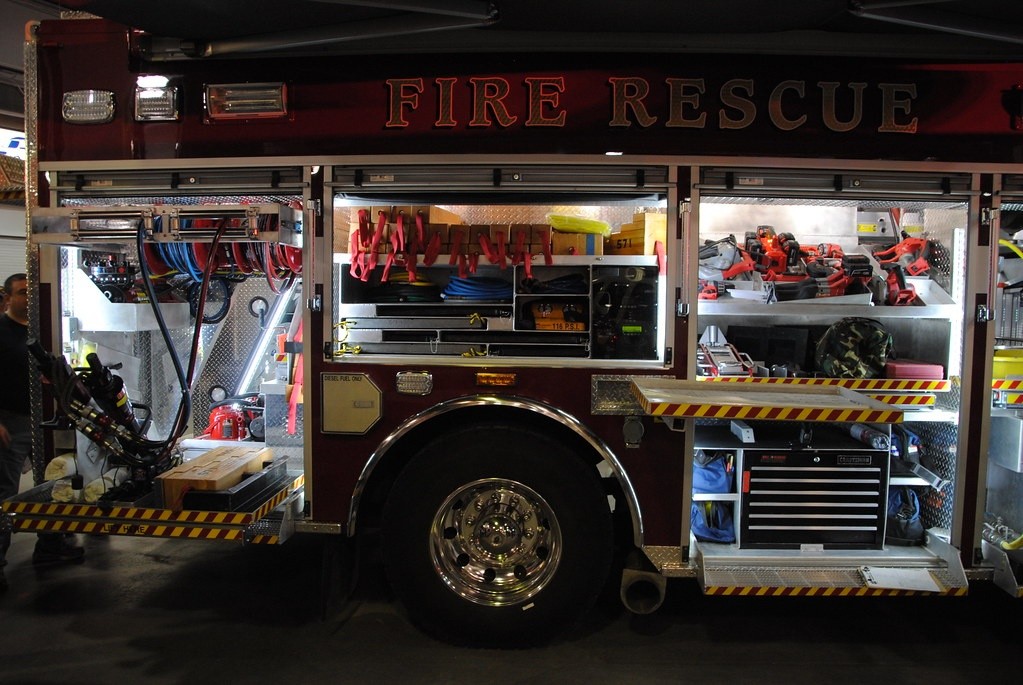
top-left (1, 0), bottom-right (1023, 654)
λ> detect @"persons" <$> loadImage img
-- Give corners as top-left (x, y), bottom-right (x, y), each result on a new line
top-left (0, 273), bottom-right (85, 565)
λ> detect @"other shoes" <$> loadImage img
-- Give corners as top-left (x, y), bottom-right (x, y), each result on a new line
top-left (33, 540), bottom-right (84, 563)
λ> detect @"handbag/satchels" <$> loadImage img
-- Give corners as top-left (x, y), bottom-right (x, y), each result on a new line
top-left (815, 317), bottom-right (894, 378)
